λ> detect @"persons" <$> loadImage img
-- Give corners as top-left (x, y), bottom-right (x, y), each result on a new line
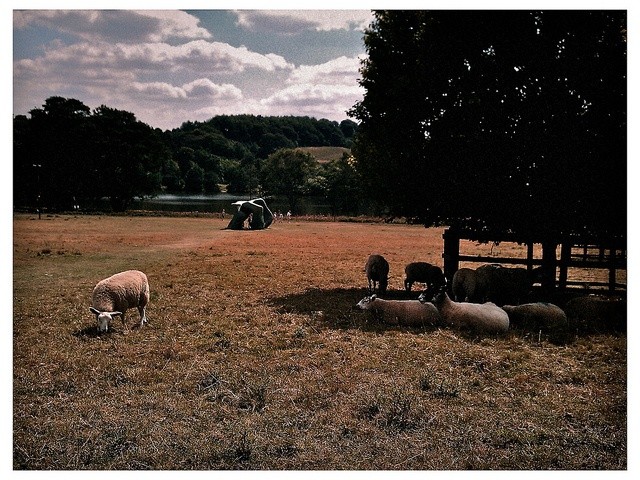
top-left (273, 212), bottom-right (277, 223)
top-left (221, 208), bottom-right (226, 221)
top-left (279, 213), bottom-right (283, 223)
top-left (242, 212), bottom-right (253, 230)
top-left (287, 210), bottom-right (291, 223)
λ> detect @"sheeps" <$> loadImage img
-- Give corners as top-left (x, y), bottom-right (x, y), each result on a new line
top-left (89, 270), bottom-right (150, 335)
top-left (365, 255), bottom-right (389, 291)
top-left (405, 262), bottom-right (446, 290)
top-left (356, 294), bottom-right (444, 326)
top-left (418, 286), bottom-right (509, 333)
top-left (501, 302), bottom-right (567, 335)
top-left (453, 264), bottom-right (543, 308)
